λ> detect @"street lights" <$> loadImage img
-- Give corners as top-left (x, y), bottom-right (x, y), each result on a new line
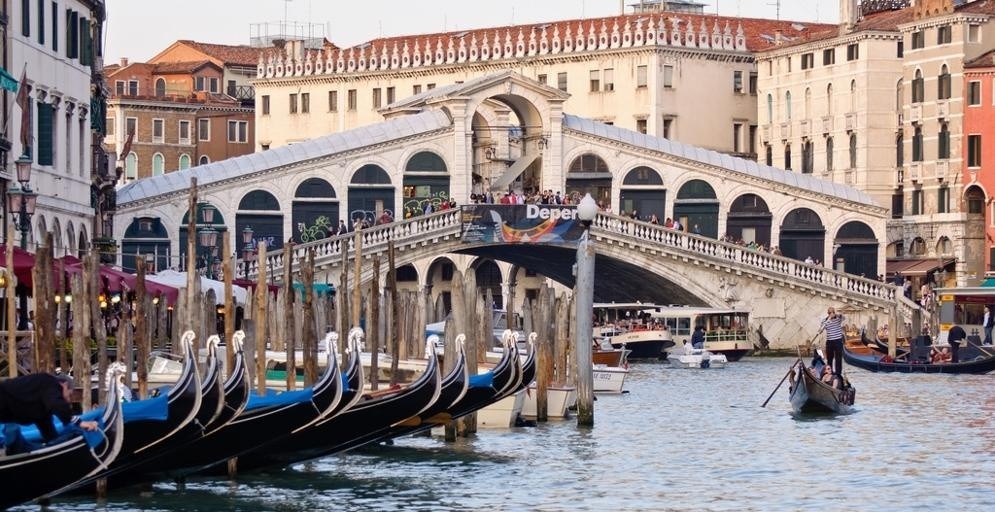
top-left (241, 224), bottom-right (254, 283)
top-left (200, 204), bottom-right (220, 280)
top-left (5, 152), bottom-right (38, 328)
top-left (576, 191), bottom-right (599, 426)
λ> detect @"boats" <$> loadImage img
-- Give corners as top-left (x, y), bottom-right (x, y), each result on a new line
top-left (844, 327), bottom-right (995, 372)
top-left (789, 349), bottom-right (857, 411)
top-left (426, 309), bottom-right (524, 350)
top-left (593, 302), bottom-right (755, 394)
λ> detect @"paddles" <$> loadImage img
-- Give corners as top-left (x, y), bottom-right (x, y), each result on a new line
top-left (761, 317), bottom-right (831, 407)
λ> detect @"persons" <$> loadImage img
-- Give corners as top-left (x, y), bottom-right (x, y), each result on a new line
top-left (4, 308), bottom-right (73, 344)
top-left (820, 365), bottom-right (844, 390)
top-left (337, 187), bottom-right (824, 267)
top-left (691, 326), bottom-right (705, 350)
top-left (592, 311), bottom-right (668, 337)
top-left (947, 318), bottom-right (967, 363)
top-left (860, 269), bottom-right (936, 311)
top-left (0, 372), bottom-right (98, 445)
top-left (817, 306), bottom-right (847, 375)
top-left (715, 322), bottom-right (727, 341)
top-left (682, 339), bottom-right (694, 355)
top-left (982, 306), bottom-right (994, 346)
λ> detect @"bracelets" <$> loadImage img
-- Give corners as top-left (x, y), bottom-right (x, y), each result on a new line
top-left (839, 314), bottom-right (843, 319)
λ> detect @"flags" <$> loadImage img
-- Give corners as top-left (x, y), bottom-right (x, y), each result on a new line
top-left (117, 129), bottom-right (135, 160)
top-left (15, 67), bottom-right (32, 157)
